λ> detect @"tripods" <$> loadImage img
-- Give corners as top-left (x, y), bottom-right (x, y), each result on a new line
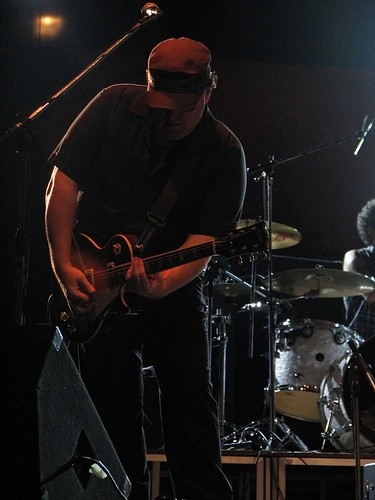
top-left (219, 120), bottom-right (375, 454)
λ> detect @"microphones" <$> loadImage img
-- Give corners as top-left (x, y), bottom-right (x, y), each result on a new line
top-left (141, 2), bottom-right (163, 16)
top-left (354, 120), bottom-right (374, 156)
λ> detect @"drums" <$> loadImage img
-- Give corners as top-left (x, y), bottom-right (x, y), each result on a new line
top-left (264, 318), bottom-right (365, 422)
top-left (315, 335), bottom-right (375, 453)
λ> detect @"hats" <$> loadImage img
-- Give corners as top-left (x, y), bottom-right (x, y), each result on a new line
top-left (145, 37), bottom-right (214, 113)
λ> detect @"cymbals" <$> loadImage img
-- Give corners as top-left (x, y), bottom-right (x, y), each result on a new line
top-left (262, 265), bottom-right (375, 298)
top-left (236, 215), bottom-right (302, 250)
top-left (205, 281), bottom-right (251, 303)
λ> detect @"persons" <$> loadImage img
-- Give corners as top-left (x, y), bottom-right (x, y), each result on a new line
top-left (44, 37), bottom-right (247, 500)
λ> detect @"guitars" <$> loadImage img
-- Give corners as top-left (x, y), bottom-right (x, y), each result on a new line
top-left (50, 216), bottom-right (266, 342)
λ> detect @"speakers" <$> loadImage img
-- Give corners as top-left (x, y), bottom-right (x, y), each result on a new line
top-left (0, 324), bottom-right (131, 500)
